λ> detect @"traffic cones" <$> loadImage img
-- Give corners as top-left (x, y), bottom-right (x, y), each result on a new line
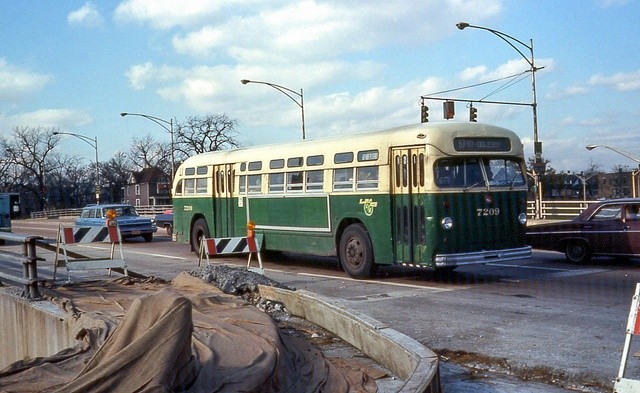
top-left (191, 224), bottom-right (274, 284)
top-left (42, 224), bottom-right (137, 294)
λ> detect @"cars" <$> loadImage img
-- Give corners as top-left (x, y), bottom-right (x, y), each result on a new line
top-left (154, 213), bottom-right (172, 234)
top-left (526, 197), bottom-right (639, 267)
top-left (75, 203), bottom-right (158, 242)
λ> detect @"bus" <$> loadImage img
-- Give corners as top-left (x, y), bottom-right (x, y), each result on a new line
top-left (170, 118), bottom-right (533, 280)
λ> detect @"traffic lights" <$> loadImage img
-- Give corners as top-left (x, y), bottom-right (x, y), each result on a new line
top-left (422, 106), bottom-right (429, 122)
top-left (469, 107), bottom-right (477, 121)
top-left (443, 101), bottom-right (455, 120)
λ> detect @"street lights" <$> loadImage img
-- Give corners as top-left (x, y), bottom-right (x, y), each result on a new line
top-left (120, 112), bottom-right (175, 190)
top-left (241, 78), bottom-right (305, 140)
top-left (456, 21), bottom-right (543, 219)
top-left (53, 131), bottom-right (101, 205)
top-left (586, 143), bottom-right (640, 166)
